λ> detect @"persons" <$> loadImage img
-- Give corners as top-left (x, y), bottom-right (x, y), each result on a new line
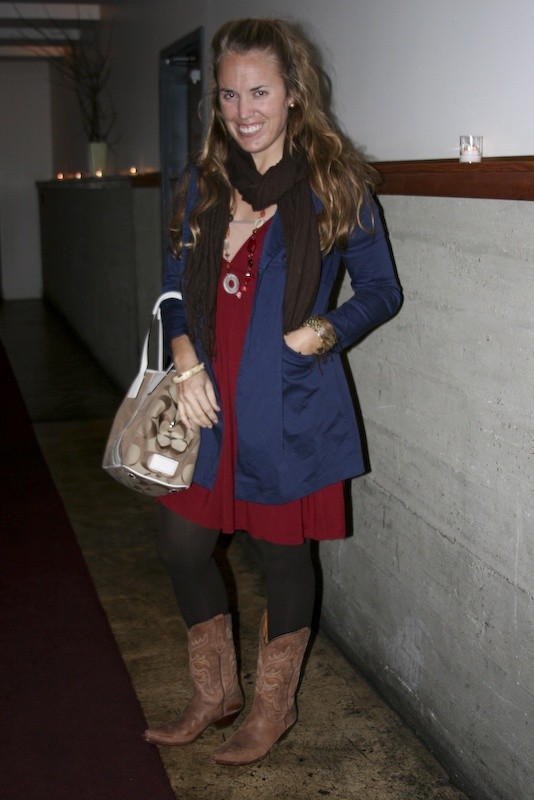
top-left (141, 20), bottom-right (405, 764)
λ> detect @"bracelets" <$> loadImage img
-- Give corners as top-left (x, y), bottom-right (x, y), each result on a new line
top-left (172, 361), bottom-right (207, 384)
top-left (301, 313), bottom-right (335, 355)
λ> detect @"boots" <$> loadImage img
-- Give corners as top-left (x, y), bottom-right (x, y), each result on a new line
top-left (143, 612), bottom-right (244, 748)
top-left (211, 608), bottom-right (311, 766)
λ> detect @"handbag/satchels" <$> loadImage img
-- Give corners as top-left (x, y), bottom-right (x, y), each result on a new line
top-left (102, 292), bottom-right (200, 493)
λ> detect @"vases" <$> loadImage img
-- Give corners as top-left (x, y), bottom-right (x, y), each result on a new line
top-left (87, 139), bottom-right (107, 176)
